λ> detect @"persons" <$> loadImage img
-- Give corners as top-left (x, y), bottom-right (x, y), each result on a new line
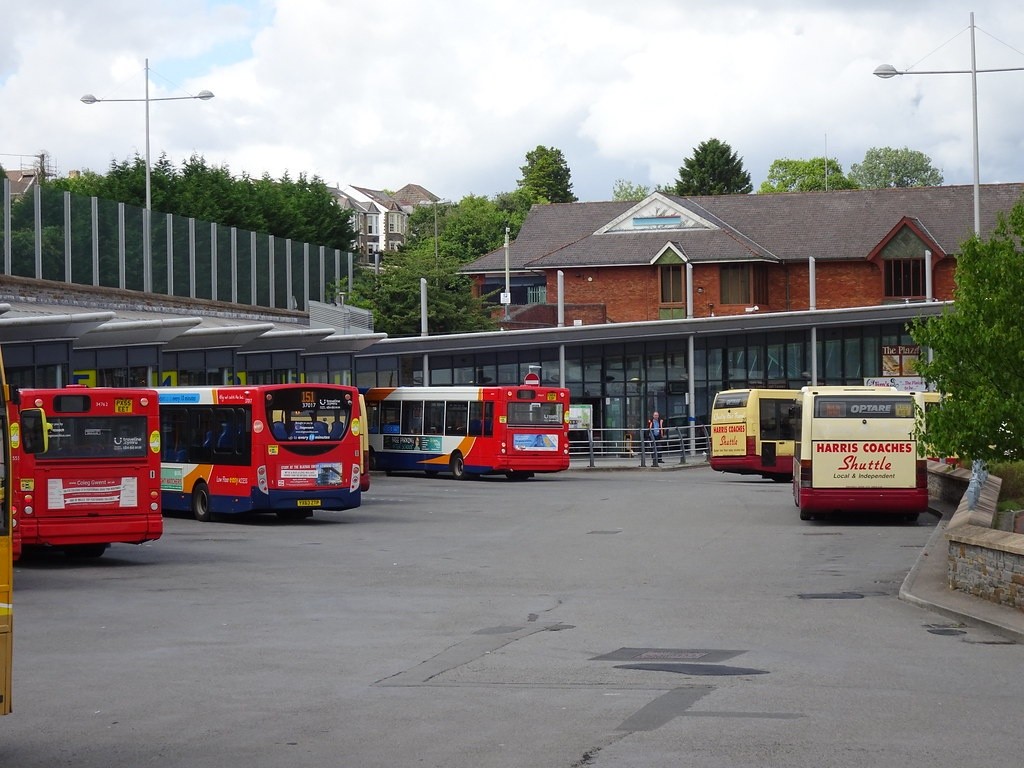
top-left (648, 411), bottom-right (665, 463)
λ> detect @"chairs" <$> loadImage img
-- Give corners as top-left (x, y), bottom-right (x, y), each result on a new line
top-left (409, 419), bottom-right (493, 436)
top-left (164, 421), bottom-right (345, 461)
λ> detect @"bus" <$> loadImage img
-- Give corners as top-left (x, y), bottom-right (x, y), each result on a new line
top-left (142, 382), bottom-right (362, 523)
top-left (272, 392), bottom-right (371, 493)
top-left (923, 391), bottom-right (1015, 468)
top-left (791, 384), bottom-right (929, 524)
top-left (705, 387), bottom-right (799, 484)
top-left (11, 383), bottom-right (167, 560)
top-left (350, 384), bottom-right (571, 482)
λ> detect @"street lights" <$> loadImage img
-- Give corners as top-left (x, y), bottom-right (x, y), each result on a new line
top-left (872, 12), bottom-right (1024, 247)
top-left (80, 58), bottom-right (217, 292)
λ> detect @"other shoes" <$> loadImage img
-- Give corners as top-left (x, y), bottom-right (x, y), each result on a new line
top-left (658, 459), bottom-right (665, 463)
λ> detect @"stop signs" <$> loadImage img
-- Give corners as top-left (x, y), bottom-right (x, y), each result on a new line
top-left (524, 374), bottom-right (541, 388)
